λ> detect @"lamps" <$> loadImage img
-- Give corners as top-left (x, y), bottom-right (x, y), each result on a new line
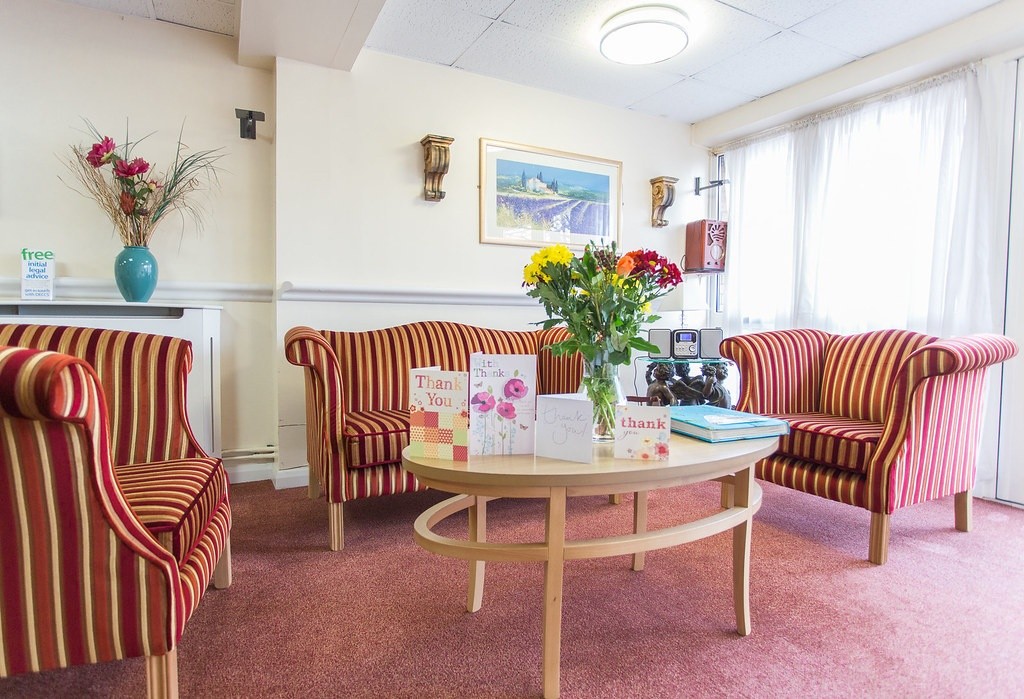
top-left (599, 6), bottom-right (691, 66)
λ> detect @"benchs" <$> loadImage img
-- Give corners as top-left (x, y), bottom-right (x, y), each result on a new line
top-left (284, 321), bottom-right (587, 551)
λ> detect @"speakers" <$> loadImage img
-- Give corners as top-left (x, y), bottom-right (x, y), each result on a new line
top-left (700, 329), bottom-right (723, 360)
top-left (648, 329), bottom-right (672, 359)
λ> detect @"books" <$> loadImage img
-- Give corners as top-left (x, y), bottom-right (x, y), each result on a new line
top-left (670, 404), bottom-right (790, 443)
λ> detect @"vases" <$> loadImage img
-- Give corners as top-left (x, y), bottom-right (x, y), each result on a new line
top-left (585, 353), bottom-right (620, 443)
top-left (115, 247), bottom-right (158, 304)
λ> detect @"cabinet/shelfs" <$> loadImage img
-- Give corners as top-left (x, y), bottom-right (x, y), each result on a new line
top-left (0, 298), bottom-right (223, 462)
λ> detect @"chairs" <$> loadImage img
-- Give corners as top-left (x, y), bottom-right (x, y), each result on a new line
top-left (719, 329), bottom-right (1019, 566)
top-left (0, 327), bottom-right (235, 699)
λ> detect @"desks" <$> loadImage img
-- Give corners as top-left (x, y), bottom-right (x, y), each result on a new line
top-left (639, 358), bottom-right (732, 407)
top-left (402, 429), bottom-right (778, 699)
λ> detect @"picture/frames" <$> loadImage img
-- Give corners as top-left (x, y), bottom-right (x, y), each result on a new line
top-left (478, 139), bottom-right (624, 257)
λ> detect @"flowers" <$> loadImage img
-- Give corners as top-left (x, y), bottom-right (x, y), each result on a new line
top-left (57, 116), bottom-right (228, 246)
top-left (522, 240), bottom-right (683, 352)
